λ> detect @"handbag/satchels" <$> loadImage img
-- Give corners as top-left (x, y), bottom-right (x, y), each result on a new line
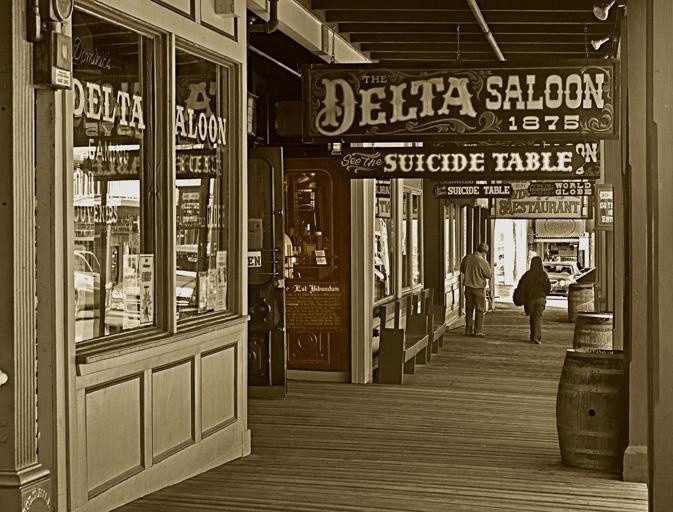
top-left (514, 288), bottom-right (525, 305)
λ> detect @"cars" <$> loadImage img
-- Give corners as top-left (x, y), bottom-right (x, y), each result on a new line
top-left (72, 239), bottom-right (218, 332)
top-left (541, 259), bottom-right (583, 297)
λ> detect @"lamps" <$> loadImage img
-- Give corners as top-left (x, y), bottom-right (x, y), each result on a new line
top-left (592, 0), bottom-right (615, 21)
top-left (590, 34), bottom-right (613, 50)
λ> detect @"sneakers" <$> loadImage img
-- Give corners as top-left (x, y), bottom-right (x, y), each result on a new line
top-left (464, 331), bottom-right (485, 337)
top-left (530, 336), bottom-right (541, 345)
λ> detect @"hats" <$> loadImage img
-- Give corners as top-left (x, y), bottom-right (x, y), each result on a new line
top-left (478, 243), bottom-right (489, 255)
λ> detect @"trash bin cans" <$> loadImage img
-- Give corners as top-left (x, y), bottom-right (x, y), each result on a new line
top-left (555, 283), bottom-right (624, 469)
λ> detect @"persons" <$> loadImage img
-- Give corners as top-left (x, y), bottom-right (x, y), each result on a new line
top-left (516, 255), bottom-right (551, 345)
top-left (459, 242), bottom-right (493, 338)
